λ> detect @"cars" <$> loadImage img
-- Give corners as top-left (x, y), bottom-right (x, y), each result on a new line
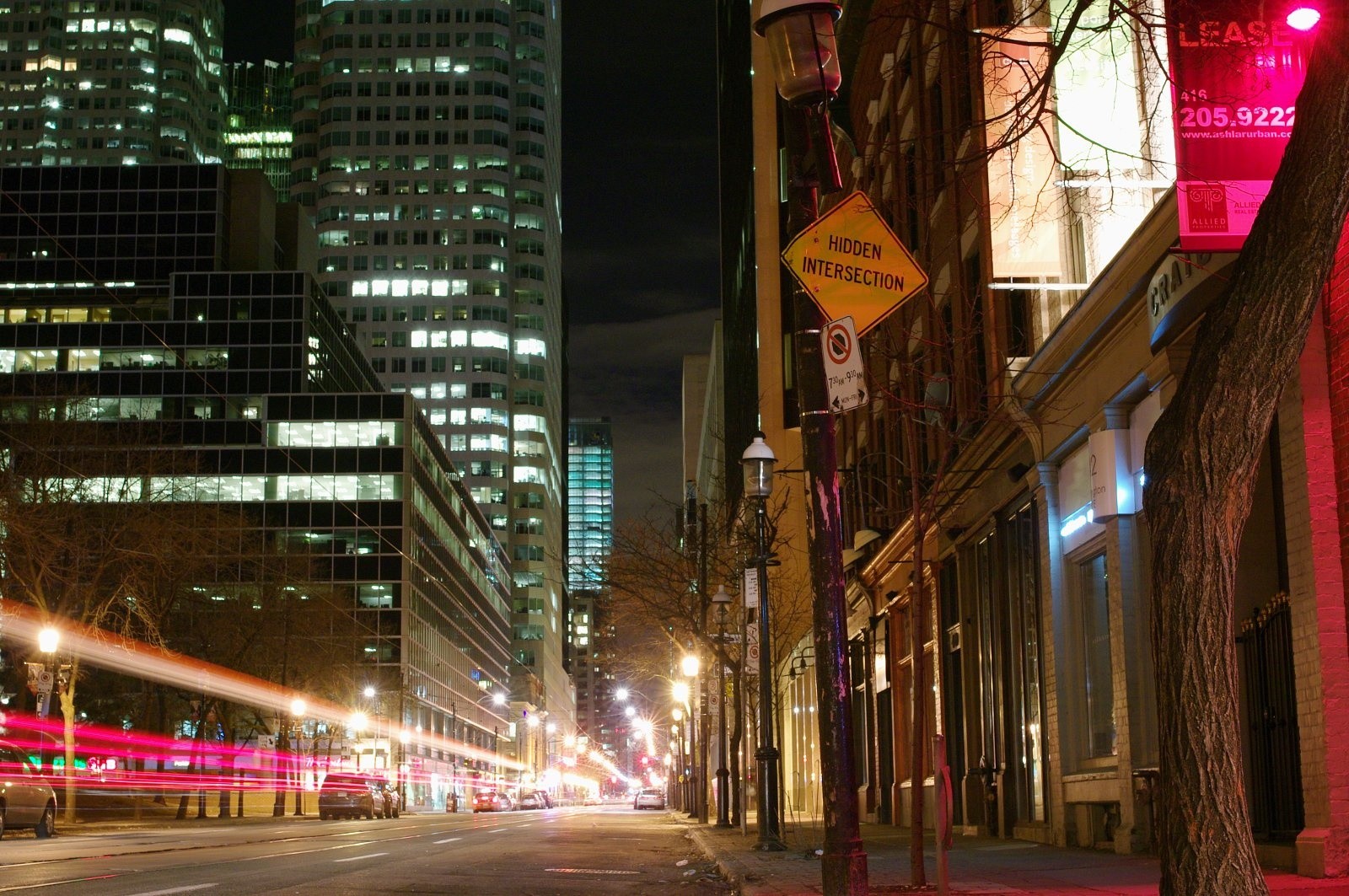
top-left (318, 772), bottom-right (401, 821)
top-left (521, 790), bottom-right (552, 809)
top-left (633, 787), bottom-right (666, 810)
top-left (472, 790), bottom-right (516, 813)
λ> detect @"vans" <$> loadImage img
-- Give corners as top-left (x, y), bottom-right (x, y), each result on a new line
top-left (0, 740), bottom-right (58, 841)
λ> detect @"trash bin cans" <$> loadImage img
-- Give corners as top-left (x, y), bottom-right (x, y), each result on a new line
top-left (446, 792), bottom-right (455, 812)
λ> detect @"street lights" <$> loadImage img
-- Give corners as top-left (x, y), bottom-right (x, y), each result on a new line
top-left (524, 714), bottom-right (540, 784)
top-left (678, 641), bottom-right (703, 826)
top-left (363, 686), bottom-right (378, 772)
top-left (290, 698), bottom-right (307, 815)
top-left (713, 584), bottom-right (740, 830)
top-left (37, 628), bottom-right (62, 786)
top-left (738, 436), bottom-right (794, 853)
top-left (666, 680), bottom-right (702, 817)
top-left (349, 712), bottom-right (368, 772)
